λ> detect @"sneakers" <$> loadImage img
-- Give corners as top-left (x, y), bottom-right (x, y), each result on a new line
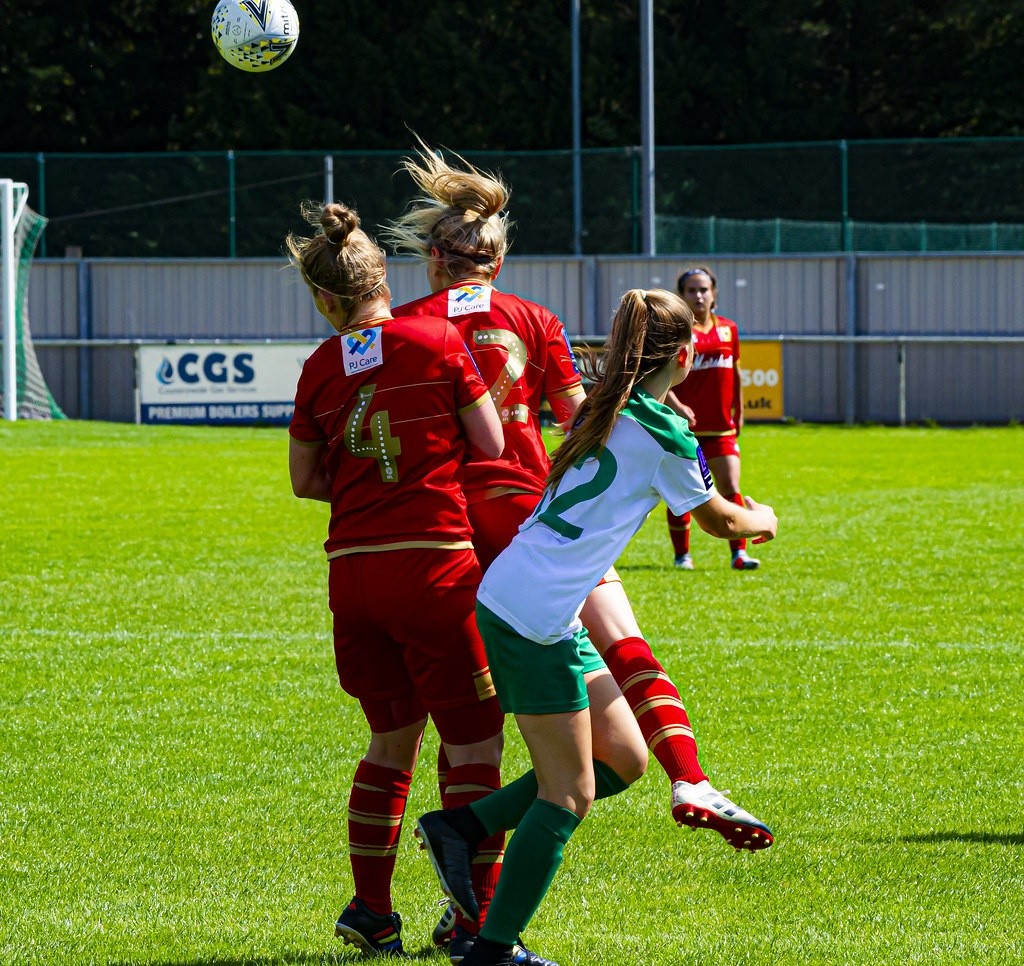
top-left (673, 553), bottom-right (692, 570)
top-left (731, 550), bottom-right (759, 570)
top-left (334, 896), bottom-right (407, 957)
top-left (449, 932), bottom-right (558, 966)
top-left (432, 903), bottom-right (488, 947)
top-left (671, 779), bottom-right (774, 853)
top-left (415, 811), bottom-right (479, 922)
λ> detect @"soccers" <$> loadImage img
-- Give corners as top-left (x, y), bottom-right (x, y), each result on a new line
top-left (210, 0), bottom-right (300, 74)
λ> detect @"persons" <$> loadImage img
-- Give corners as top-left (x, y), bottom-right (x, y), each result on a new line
top-left (289, 202), bottom-right (561, 966)
top-left (378, 134), bottom-right (773, 947)
top-left (415, 289), bottom-right (778, 966)
top-left (668, 267), bottom-right (762, 570)
